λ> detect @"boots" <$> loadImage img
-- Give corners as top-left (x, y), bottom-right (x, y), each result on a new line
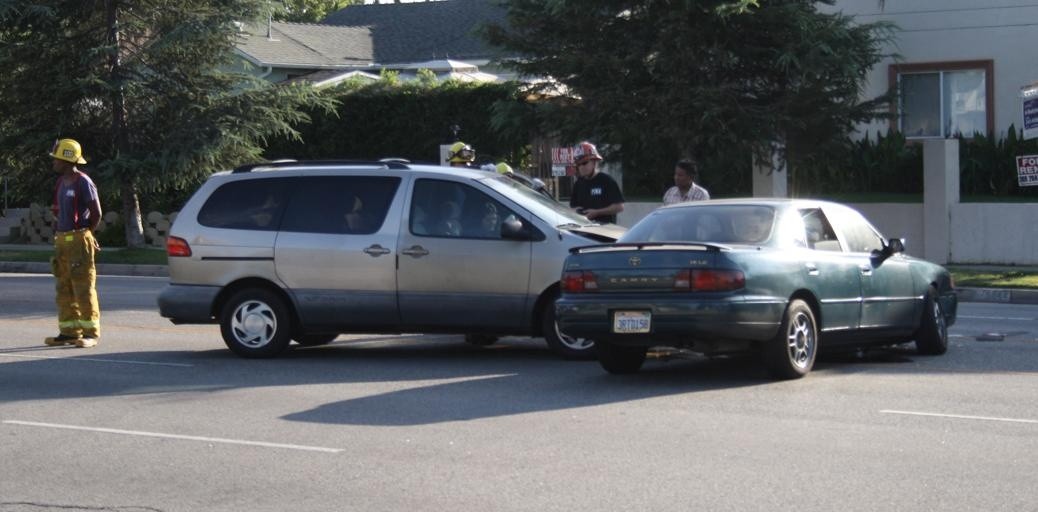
top-left (44, 333), bottom-right (83, 346)
top-left (75, 336), bottom-right (99, 348)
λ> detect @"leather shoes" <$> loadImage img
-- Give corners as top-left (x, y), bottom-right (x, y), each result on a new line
top-left (470, 332), bottom-right (484, 345)
top-left (464, 331), bottom-right (471, 343)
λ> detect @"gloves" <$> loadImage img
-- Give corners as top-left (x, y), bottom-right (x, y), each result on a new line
top-left (479, 162), bottom-right (495, 172)
top-left (530, 178), bottom-right (545, 191)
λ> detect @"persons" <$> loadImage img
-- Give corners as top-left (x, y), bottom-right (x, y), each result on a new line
top-left (446, 142), bottom-right (494, 348)
top-left (43, 138), bottom-right (102, 349)
top-left (662, 159), bottom-right (709, 205)
top-left (492, 161), bottom-right (512, 177)
top-left (569, 143), bottom-right (625, 225)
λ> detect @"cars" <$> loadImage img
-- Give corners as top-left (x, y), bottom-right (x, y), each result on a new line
top-left (156, 156), bottom-right (631, 361)
top-left (553, 196), bottom-right (958, 380)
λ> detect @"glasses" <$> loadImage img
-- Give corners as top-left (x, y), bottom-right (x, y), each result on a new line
top-left (576, 160), bottom-right (589, 166)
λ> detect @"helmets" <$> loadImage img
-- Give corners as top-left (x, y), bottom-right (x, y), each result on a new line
top-left (494, 162), bottom-right (514, 174)
top-left (446, 142), bottom-right (476, 163)
top-left (48, 137), bottom-right (88, 166)
top-left (573, 142), bottom-right (603, 167)
